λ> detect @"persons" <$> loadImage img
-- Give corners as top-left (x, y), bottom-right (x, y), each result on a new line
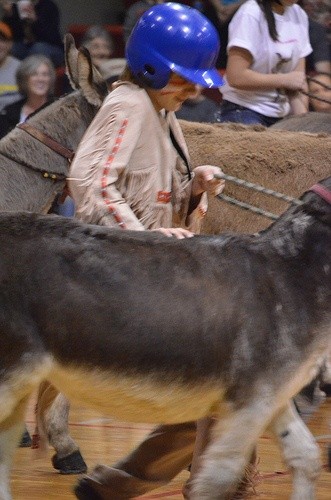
top-left (0, 0), bottom-right (114, 216)
top-left (175, 0), bottom-right (331, 125)
top-left (67, 2), bottom-right (257, 500)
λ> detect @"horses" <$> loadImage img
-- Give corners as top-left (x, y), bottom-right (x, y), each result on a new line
top-left (1, 110), bottom-right (331, 500)
top-left (0, 35), bottom-right (121, 471)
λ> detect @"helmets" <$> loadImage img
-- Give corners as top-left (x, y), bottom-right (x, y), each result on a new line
top-left (125, 3), bottom-right (226, 89)
